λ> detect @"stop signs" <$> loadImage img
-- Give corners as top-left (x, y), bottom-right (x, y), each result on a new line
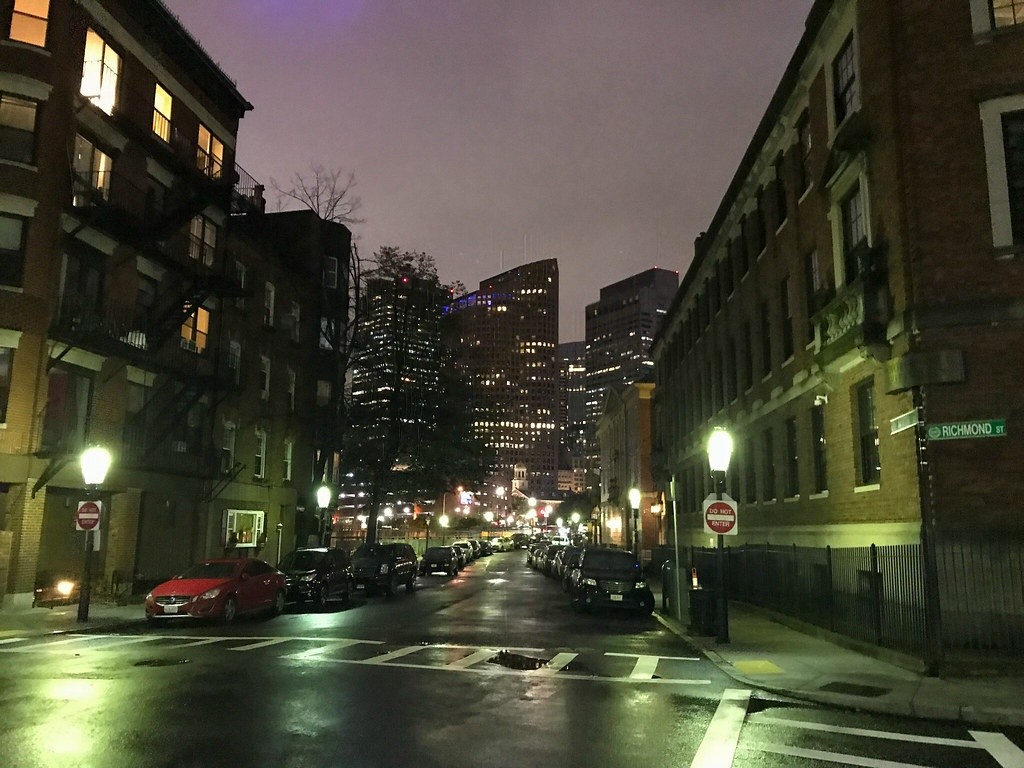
top-left (75, 501), bottom-right (102, 531)
top-left (703, 499), bottom-right (738, 535)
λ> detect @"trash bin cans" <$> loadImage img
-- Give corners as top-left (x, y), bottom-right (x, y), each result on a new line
top-left (690, 589), bottom-right (717, 636)
top-left (662, 559), bottom-right (691, 618)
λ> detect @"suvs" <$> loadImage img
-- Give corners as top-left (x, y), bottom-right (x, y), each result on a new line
top-left (346, 541), bottom-right (418, 598)
top-left (275, 546), bottom-right (354, 606)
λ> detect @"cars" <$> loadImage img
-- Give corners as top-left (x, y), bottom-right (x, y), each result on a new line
top-left (419, 538), bottom-right (514, 576)
top-left (552, 544), bottom-right (656, 615)
top-left (145, 559), bottom-right (288, 626)
top-left (512, 534), bottom-right (570, 574)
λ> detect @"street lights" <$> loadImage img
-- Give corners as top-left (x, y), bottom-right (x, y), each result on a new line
top-left (484, 510), bottom-right (494, 541)
top-left (75, 443), bottom-right (113, 623)
top-left (708, 422), bottom-right (734, 646)
top-left (626, 485), bottom-right (642, 555)
top-left (571, 511), bottom-right (580, 541)
top-left (439, 512), bottom-right (450, 545)
top-left (316, 483), bottom-right (331, 548)
